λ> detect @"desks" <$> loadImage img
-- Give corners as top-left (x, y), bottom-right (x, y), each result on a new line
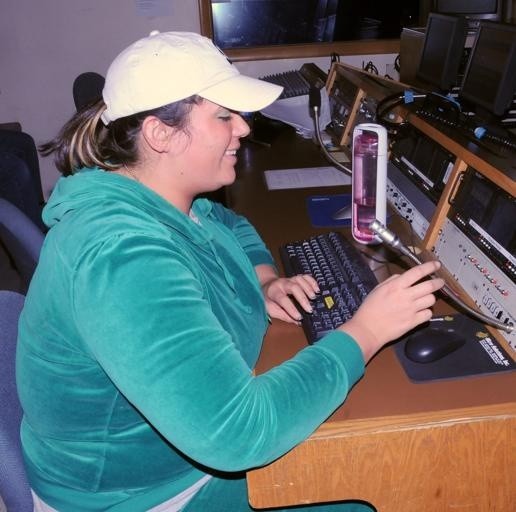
top-left (223, 115), bottom-right (516, 511)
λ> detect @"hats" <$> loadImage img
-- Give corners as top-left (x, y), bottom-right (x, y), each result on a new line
top-left (100, 29), bottom-right (286, 127)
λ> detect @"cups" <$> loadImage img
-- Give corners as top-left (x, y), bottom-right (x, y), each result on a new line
top-left (350, 122), bottom-right (389, 245)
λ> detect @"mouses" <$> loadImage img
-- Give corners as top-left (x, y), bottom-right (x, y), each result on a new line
top-left (331, 203), bottom-right (352, 221)
top-left (404, 326), bottom-right (467, 363)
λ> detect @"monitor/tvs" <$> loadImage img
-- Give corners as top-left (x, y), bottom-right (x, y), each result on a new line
top-left (415, 13), bottom-right (468, 91)
top-left (458, 20), bottom-right (516, 116)
top-left (434, 0), bottom-right (513, 29)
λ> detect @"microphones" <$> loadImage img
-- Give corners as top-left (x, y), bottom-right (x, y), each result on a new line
top-left (308, 87), bottom-right (352, 174)
top-left (369, 218), bottom-right (513, 333)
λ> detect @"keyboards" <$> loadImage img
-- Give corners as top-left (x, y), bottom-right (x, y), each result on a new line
top-left (278, 229), bottom-right (380, 346)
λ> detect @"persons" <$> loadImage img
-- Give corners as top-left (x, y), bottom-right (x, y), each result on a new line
top-left (11, 28), bottom-right (444, 512)
